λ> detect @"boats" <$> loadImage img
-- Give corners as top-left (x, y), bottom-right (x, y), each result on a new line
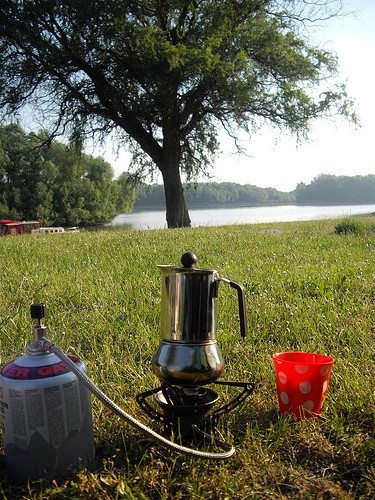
top-left (30, 226), bottom-right (84, 235)
top-left (1, 219), bottom-right (40, 235)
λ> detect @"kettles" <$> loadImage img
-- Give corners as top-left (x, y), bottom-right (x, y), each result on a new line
top-left (155, 252), bottom-right (249, 344)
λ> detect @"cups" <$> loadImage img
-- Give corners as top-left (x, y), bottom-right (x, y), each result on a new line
top-left (272, 352), bottom-right (334, 422)
